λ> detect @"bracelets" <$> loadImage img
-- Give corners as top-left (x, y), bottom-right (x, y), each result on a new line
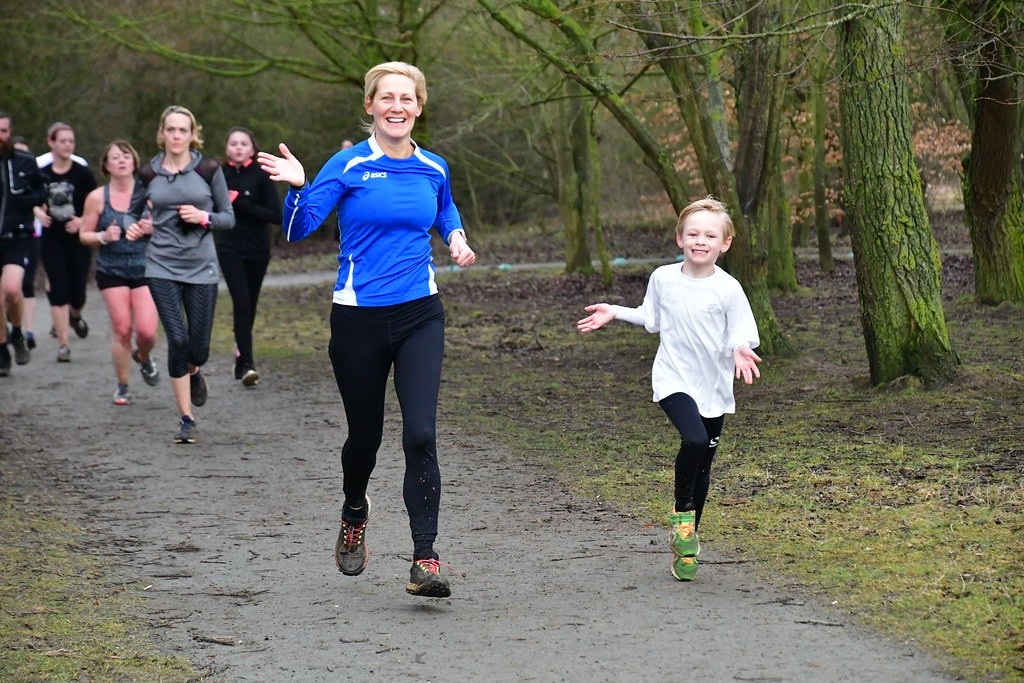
top-left (290, 172), bottom-right (307, 191)
top-left (209, 211), bottom-right (211, 229)
top-left (98, 229), bottom-right (108, 246)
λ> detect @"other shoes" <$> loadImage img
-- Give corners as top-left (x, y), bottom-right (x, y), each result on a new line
top-left (0, 347), bottom-right (12, 375)
top-left (25, 332), bottom-right (36, 349)
top-left (11, 330), bottom-right (31, 365)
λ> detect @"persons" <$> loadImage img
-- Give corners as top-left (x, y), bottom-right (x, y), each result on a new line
top-left (210, 125), bottom-right (283, 386)
top-left (0, 113), bottom-right (97, 377)
top-left (122, 105), bottom-right (237, 442)
top-left (77, 139), bottom-right (158, 404)
top-left (257, 61), bottom-right (479, 597)
top-left (576, 195), bottom-right (764, 582)
top-left (335, 128), bottom-right (425, 247)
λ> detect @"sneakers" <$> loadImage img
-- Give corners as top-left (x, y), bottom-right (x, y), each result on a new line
top-left (190, 366), bottom-right (208, 407)
top-left (336, 495), bottom-right (369, 576)
top-left (242, 370), bottom-right (258, 387)
top-left (405, 557), bottom-right (451, 597)
top-left (671, 551), bottom-right (699, 581)
top-left (57, 345), bottom-right (70, 362)
top-left (114, 382), bottom-right (131, 405)
top-left (69, 313), bottom-right (89, 339)
top-left (132, 347), bottom-right (161, 386)
top-left (174, 415), bottom-right (196, 443)
top-left (232, 342), bottom-right (243, 379)
top-left (668, 499), bottom-right (700, 556)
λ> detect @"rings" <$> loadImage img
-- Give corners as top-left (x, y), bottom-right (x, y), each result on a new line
top-left (465, 265), bottom-right (468, 267)
